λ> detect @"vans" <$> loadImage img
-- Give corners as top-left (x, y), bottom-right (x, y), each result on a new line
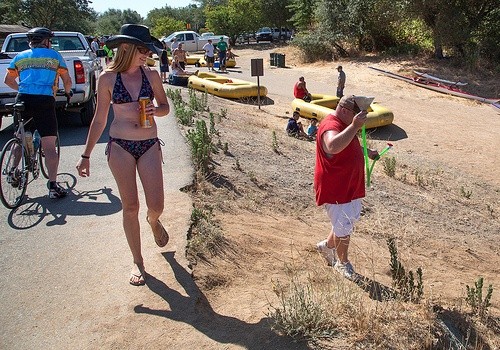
top-left (0, 25), bottom-right (30, 52)
top-left (271, 27), bottom-right (295, 41)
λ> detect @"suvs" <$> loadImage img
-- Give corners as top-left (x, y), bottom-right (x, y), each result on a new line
top-left (254, 27), bottom-right (275, 43)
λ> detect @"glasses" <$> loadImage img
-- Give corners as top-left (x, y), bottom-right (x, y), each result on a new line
top-left (136, 47), bottom-right (150, 54)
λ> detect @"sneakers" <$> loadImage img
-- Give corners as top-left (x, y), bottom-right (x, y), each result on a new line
top-left (8, 169), bottom-right (19, 188)
top-left (334, 260), bottom-right (359, 283)
top-left (50, 188), bottom-right (66, 198)
top-left (317, 239), bottom-right (336, 265)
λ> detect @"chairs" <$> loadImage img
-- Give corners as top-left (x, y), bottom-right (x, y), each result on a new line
top-left (64, 40), bottom-right (76, 50)
top-left (16, 41), bottom-right (29, 51)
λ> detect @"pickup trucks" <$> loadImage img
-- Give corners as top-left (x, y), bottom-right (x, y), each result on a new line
top-left (0, 30), bottom-right (108, 129)
top-left (160, 31), bottom-right (230, 53)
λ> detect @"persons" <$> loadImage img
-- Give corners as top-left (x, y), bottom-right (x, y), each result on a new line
top-left (172, 42), bottom-right (187, 70)
top-left (159, 48), bottom-right (169, 84)
top-left (216, 37), bottom-right (229, 71)
top-left (308, 118), bottom-right (318, 136)
top-left (103, 44), bottom-right (113, 62)
top-left (286, 111), bottom-right (313, 142)
top-left (314, 93), bottom-right (380, 283)
top-left (75, 24), bottom-right (170, 285)
top-left (202, 40), bottom-right (215, 72)
top-left (4, 27), bottom-right (72, 199)
top-left (336, 66), bottom-right (346, 97)
top-left (294, 77), bottom-right (312, 103)
top-left (171, 39), bottom-right (178, 50)
top-left (171, 55), bottom-right (199, 76)
top-left (91, 37), bottom-right (99, 52)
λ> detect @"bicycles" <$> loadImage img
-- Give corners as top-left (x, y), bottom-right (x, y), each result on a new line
top-left (0, 89), bottom-right (74, 210)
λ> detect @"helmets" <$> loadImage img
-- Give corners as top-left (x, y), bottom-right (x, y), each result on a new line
top-left (27, 27), bottom-right (54, 40)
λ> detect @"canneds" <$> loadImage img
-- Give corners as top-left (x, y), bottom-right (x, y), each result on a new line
top-left (139, 96), bottom-right (153, 128)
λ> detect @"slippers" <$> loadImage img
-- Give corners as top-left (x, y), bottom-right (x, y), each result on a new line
top-left (130, 271), bottom-right (145, 286)
top-left (146, 216), bottom-right (169, 247)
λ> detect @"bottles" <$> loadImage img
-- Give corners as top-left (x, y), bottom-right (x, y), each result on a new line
top-left (33, 130), bottom-right (39, 149)
top-left (25, 132), bottom-right (34, 155)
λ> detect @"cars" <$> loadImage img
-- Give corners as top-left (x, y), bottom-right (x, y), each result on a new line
top-left (83, 35), bottom-right (110, 48)
top-left (201, 32), bottom-right (215, 37)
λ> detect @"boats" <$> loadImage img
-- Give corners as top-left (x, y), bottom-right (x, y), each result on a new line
top-left (291, 93), bottom-right (394, 132)
top-left (187, 71), bottom-right (268, 99)
top-left (168, 56), bottom-right (201, 65)
top-left (198, 57), bottom-right (236, 68)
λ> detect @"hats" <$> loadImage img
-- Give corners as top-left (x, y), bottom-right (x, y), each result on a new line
top-left (106, 24), bottom-right (164, 50)
top-left (336, 66), bottom-right (342, 69)
top-left (339, 94), bottom-right (362, 114)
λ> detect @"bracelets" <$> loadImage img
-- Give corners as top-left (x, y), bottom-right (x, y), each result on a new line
top-left (81, 154), bottom-right (89, 159)
